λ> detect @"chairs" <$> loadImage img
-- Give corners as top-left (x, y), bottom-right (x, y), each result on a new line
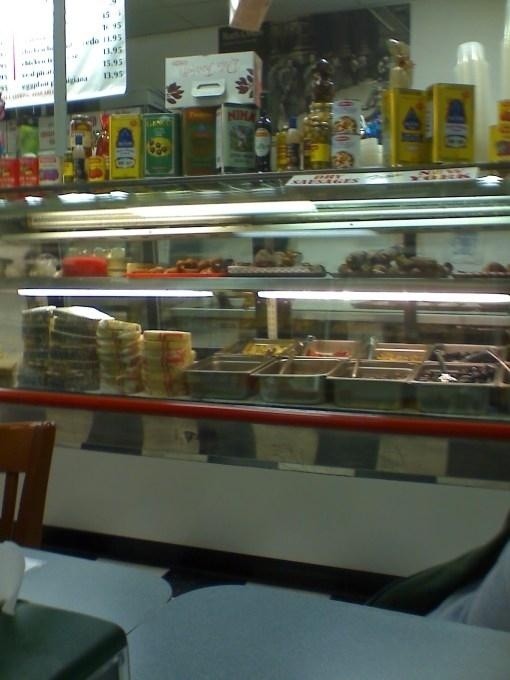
top-left (1, 420), bottom-right (57, 550)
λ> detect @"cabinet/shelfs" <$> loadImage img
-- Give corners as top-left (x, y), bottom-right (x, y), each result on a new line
top-left (0, 161), bottom-right (509, 579)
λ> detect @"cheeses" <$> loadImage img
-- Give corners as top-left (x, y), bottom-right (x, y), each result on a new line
top-left (16, 305), bottom-right (198, 398)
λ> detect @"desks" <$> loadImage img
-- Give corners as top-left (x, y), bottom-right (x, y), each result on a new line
top-left (127, 583), bottom-right (510, 680)
top-left (0, 541), bottom-right (173, 680)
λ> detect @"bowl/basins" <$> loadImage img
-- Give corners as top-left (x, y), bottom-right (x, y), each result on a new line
top-left (228, 297), bottom-right (245, 308)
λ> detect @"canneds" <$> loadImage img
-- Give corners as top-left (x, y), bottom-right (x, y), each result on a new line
top-left (1, 114), bottom-right (105, 189)
top-left (303, 96), bottom-right (361, 168)
top-left (487, 99), bottom-right (510, 164)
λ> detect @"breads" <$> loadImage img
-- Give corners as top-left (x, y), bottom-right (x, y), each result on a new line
top-left (482, 262), bottom-right (506, 272)
top-left (176, 248), bottom-right (303, 274)
top-left (338, 242), bottom-right (454, 276)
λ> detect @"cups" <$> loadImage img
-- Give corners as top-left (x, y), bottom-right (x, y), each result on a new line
top-left (455, 41), bottom-right (495, 165)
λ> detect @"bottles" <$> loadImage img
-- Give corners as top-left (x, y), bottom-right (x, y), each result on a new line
top-left (63, 135), bottom-right (86, 187)
top-left (255, 91), bottom-right (300, 169)
top-left (107, 246), bottom-right (127, 277)
top-left (16, 117), bottom-right (38, 162)
